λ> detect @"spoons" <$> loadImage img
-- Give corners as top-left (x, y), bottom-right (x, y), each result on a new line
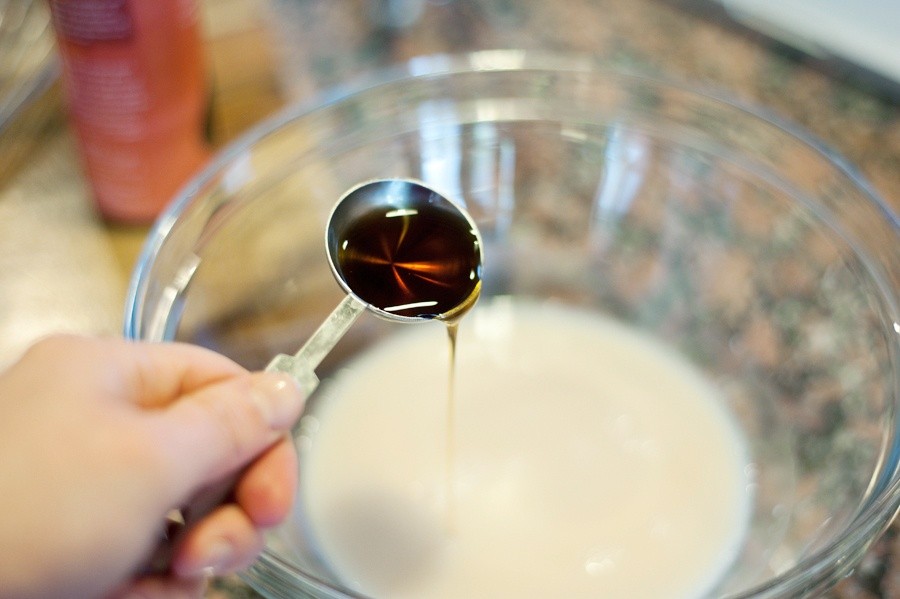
top-left (262, 180), bottom-right (482, 396)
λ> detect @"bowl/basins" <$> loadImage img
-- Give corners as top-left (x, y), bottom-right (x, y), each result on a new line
top-left (116, 57), bottom-right (900, 599)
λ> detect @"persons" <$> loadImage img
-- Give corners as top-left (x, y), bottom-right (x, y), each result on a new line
top-left (0, 332), bottom-right (306, 599)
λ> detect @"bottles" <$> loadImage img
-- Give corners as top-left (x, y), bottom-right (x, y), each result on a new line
top-left (46, 0), bottom-right (224, 293)
